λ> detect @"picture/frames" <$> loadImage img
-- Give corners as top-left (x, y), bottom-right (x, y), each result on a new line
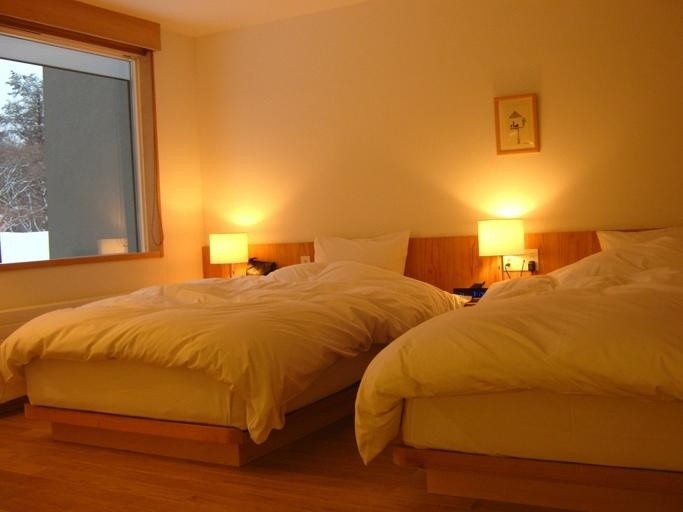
top-left (493, 92), bottom-right (541, 157)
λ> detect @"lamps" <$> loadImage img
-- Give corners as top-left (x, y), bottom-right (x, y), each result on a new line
top-left (209, 232), bottom-right (248, 279)
top-left (476, 218), bottom-right (525, 282)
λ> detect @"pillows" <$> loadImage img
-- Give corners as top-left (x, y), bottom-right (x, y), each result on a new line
top-left (594, 225), bottom-right (683, 252)
top-left (314, 229), bottom-right (410, 277)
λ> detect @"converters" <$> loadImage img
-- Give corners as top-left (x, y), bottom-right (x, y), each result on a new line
top-left (528, 261), bottom-right (535, 271)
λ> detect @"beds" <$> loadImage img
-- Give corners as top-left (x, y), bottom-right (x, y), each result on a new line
top-left (391, 279), bottom-right (682, 511)
top-left (22, 279), bottom-right (452, 466)
